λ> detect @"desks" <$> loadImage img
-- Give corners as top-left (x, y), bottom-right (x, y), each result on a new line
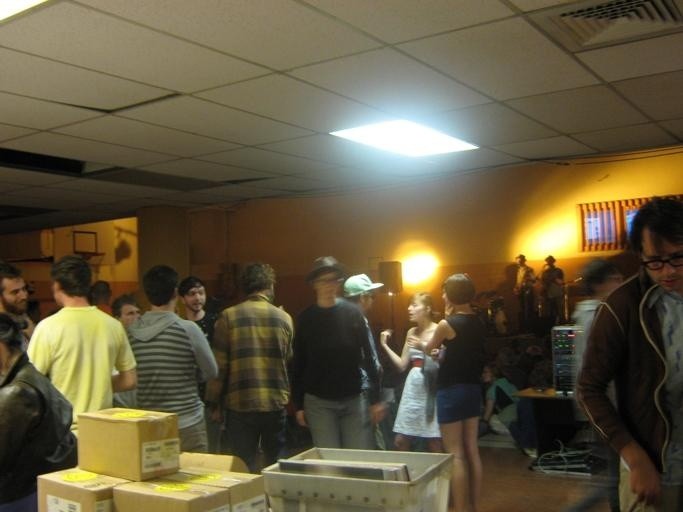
top-left (510, 385), bottom-right (573, 456)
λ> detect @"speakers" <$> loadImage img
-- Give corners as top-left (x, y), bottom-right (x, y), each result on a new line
top-left (378, 260), bottom-right (403, 293)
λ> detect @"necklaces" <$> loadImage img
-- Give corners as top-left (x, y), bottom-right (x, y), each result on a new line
top-left (570, 193), bottom-right (682, 511)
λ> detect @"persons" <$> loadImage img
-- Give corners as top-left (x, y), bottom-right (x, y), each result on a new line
top-left (1, 252), bottom-right (572, 512)
top-left (567, 252), bottom-right (625, 511)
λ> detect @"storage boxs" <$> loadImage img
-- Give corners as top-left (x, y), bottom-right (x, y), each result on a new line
top-left (35, 465), bottom-right (133, 512)
top-left (112, 482), bottom-right (228, 512)
top-left (76, 406), bottom-right (181, 483)
top-left (158, 467), bottom-right (266, 512)
top-left (259, 444), bottom-right (453, 512)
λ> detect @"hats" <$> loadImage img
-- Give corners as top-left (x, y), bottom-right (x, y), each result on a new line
top-left (344, 273), bottom-right (384, 297)
top-left (305, 256), bottom-right (340, 280)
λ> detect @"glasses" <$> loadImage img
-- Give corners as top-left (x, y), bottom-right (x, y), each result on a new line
top-left (638, 254), bottom-right (683, 270)
